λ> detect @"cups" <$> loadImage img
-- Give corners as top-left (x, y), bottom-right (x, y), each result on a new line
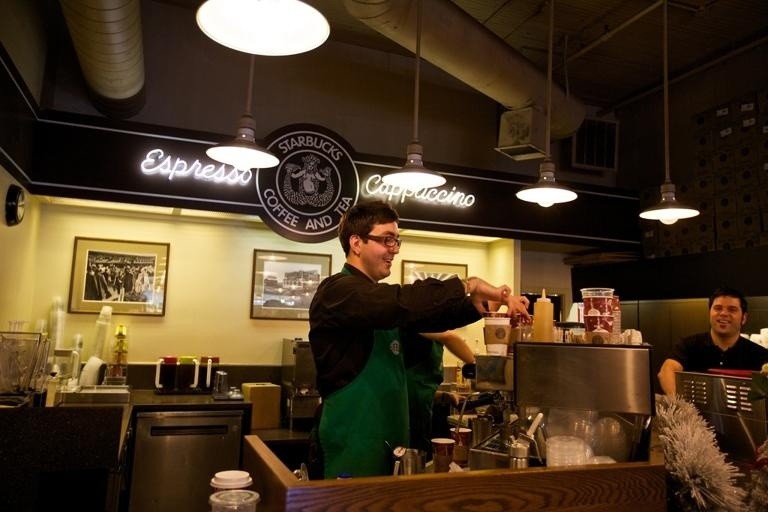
top-left (207, 468), bottom-right (261, 511)
top-left (35, 296), bottom-right (83, 352)
top-left (581, 286), bottom-right (622, 345)
top-left (215, 371), bottom-right (228, 398)
top-left (432, 427), bottom-right (471, 472)
top-left (483, 312), bottom-right (511, 356)
top-left (156, 355), bottom-right (219, 394)
top-left (469, 418), bottom-right (489, 438)
top-left (90, 305), bottom-right (112, 359)
top-left (392, 449), bottom-right (427, 475)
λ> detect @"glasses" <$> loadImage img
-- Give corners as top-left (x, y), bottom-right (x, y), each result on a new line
top-left (365, 234), bottom-right (401, 247)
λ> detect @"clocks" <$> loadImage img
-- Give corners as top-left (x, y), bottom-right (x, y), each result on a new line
top-left (6, 183), bottom-right (25, 226)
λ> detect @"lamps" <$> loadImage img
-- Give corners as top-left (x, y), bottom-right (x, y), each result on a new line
top-left (515, 1), bottom-right (577, 209)
top-left (204, 56), bottom-right (280, 173)
top-left (380, 0), bottom-right (448, 192)
top-left (639, 1), bottom-right (701, 225)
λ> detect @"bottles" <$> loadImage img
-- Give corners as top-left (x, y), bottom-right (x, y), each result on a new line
top-left (533, 288), bottom-right (553, 342)
top-left (228, 386), bottom-right (243, 398)
top-left (33, 349), bottom-right (79, 408)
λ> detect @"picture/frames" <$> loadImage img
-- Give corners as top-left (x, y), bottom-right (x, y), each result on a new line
top-left (401, 258), bottom-right (469, 291)
top-left (67, 236), bottom-right (170, 316)
top-left (249, 247), bottom-right (333, 321)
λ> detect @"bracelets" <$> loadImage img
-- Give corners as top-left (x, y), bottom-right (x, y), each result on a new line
top-left (471, 356), bottom-right (477, 365)
top-left (463, 278), bottom-right (472, 295)
top-left (441, 390), bottom-right (445, 402)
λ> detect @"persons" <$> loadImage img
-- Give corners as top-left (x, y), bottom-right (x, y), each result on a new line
top-left (308, 199), bottom-right (531, 478)
top-left (657, 285), bottom-right (766, 397)
top-left (399, 277), bottom-right (476, 462)
top-left (85, 262), bottom-right (155, 302)
